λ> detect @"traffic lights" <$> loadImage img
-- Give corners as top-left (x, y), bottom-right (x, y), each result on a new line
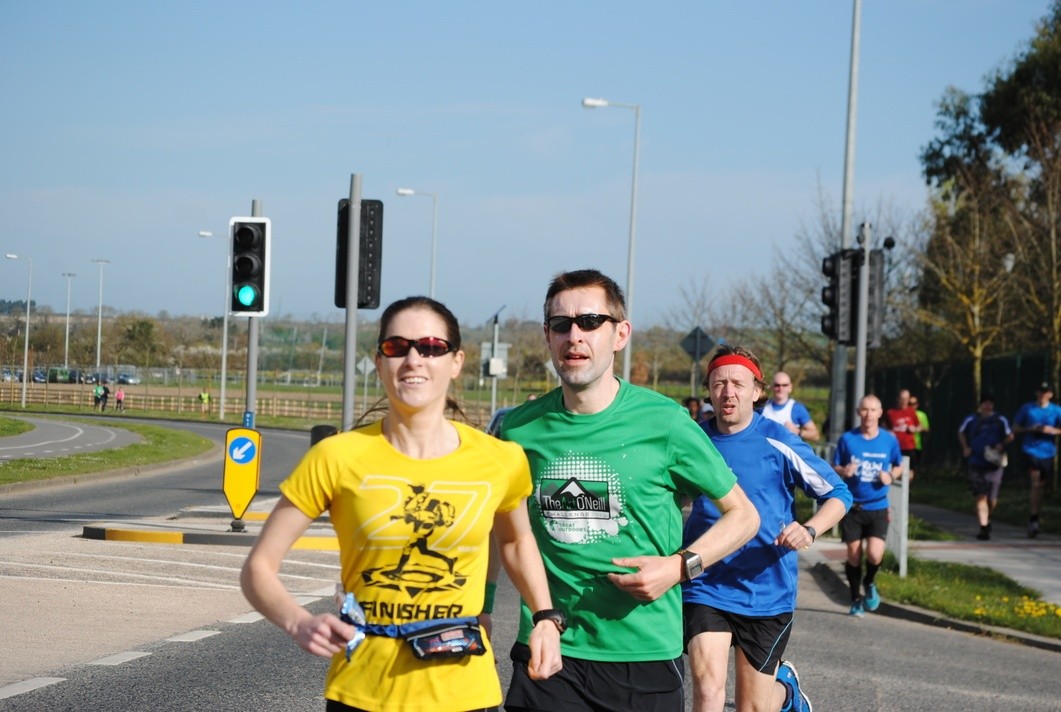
top-left (821, 248), bottom-right (861, 346)
top-left (225, 216), bottom-right (271, 317)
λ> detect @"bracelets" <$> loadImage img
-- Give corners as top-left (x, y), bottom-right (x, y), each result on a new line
top-left (799, 427), bottom-right (801, 436)
top-left (888, 472), bottom-right (894, 484)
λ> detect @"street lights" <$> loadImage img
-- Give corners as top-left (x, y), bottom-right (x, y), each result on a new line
top-left (61, 272), bottom-right (78, 368)
top-left (199, 229), bottom-right (233, 419)
top-left (5, 253), bottom-right (33, 408)
top-left (94, 259), bottom-right (108, 373)
top-left (396, 188), bottom-right (440, 301)
top-left (583, 97), bottom-right (642, 383)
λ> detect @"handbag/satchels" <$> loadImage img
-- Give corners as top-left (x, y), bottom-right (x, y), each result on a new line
top-left (405, 618), bottom-right (488, 660)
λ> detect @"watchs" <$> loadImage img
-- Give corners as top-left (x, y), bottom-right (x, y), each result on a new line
top-left (531, 608), bottom-right (567, 634)
top-left (677, 549), bottom-right (704, 581)
top-left (803, 525), bottom-right (816, 543)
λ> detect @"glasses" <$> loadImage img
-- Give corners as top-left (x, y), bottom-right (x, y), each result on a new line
top-left (546, 313), bottom-right (620, 334)
top-left (378, 336), bottom-right (459, 358)
top-left (774, 383), bottom-right (790, 388)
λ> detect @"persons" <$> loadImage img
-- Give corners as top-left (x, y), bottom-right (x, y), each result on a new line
top-left (93, 377), bottom-right (126, 414)
top-left (829, 394), bottom-right (905, 617)
top-left (957, 394), bottom-right (1014, 541)
top-left (1013, 380), bottom-right (1061, 538)
top-left (199, 386), bottom-right (211, 417)
top-left (239, 296), bottom-right (569, 712)
top-left (528, 393), bottom-right (538, 401)
top-left (476, 268), bottom-right (761, 712)
top-left (753, 370), bottom-right (820, 444)
top-left (681, 396), bottom-right (715, 426)
top-left (880, 387), bottom-right (930, 483)
top-left (679, 342), bottom-right (855, 712)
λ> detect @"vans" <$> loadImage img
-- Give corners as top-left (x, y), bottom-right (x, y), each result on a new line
top-left (48, 366), bottom-right (69, 383)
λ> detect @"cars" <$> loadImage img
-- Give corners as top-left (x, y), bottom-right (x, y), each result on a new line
top-left (31, 367), bottom-right (47, 383)
top-left (69, 369), bottom-right (141, 385)
top-left (2, 368), bottom-right (21, 383)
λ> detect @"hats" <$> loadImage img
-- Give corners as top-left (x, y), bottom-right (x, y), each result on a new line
top-left (1035, 381), bottom-right (1052, 393)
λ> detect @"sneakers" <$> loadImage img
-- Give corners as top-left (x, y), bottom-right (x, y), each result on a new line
top-left (863, 576), bottom-right (879, 611)
top-left (775, 660), bottom-right (813, 712)
top-left (850, 597), bottom-right (864, 618)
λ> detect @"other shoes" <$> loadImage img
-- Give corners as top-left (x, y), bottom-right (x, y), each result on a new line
top-left (976, 524), bottom-right (991, 540)
top-left (1027, 522), bottom-right (1039, 537)
